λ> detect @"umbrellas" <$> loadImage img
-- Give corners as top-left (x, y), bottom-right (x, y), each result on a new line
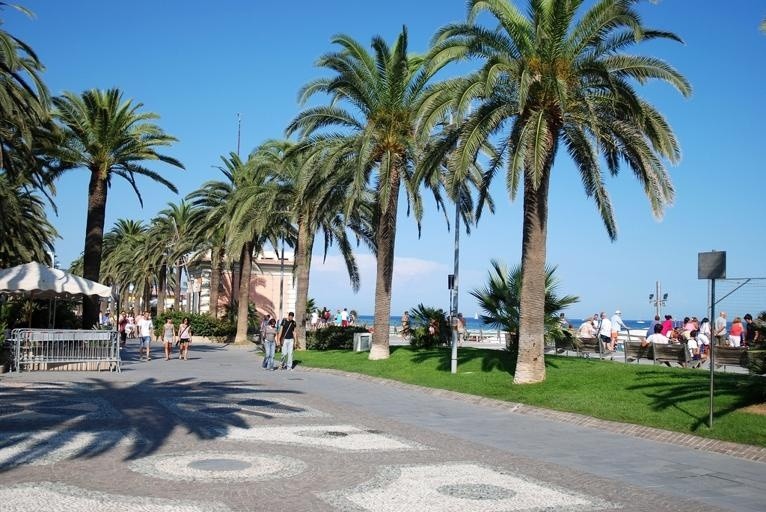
top-left (0, 261), bottom-right (112, 328)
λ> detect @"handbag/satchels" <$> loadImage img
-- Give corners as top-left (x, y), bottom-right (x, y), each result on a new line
top-left (280, 336), bottom-right (284, 345)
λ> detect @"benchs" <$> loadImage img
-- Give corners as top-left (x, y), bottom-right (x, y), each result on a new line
top-left (710, 344), bottom-right (747, 372)
top-left (624, 341), bottom-right (690, 369)
top-left (555, 337), bottom-right (605, 360)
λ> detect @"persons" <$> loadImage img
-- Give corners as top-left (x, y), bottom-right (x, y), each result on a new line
top-left (428, 310), bottom-right (464, 344)
top-left (100, 309), bottom-right (191, 360)
top-left (310, 307), bottom-right (356, 331)
top-left (641, 312), bottom-right (760, 368)
top-left (260, 313), bottom-right (297, 373)
top-left (554, 309), bottom-right (629, 356)
top-left (401, 311), bottom-right (410, 339)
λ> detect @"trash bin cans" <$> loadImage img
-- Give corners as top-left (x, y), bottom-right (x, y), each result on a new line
top-left (253, 334), bottom-right (260, 342)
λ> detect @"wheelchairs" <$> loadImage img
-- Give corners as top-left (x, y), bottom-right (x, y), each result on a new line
top-left (399, 320), bottom-right (415, 342)
